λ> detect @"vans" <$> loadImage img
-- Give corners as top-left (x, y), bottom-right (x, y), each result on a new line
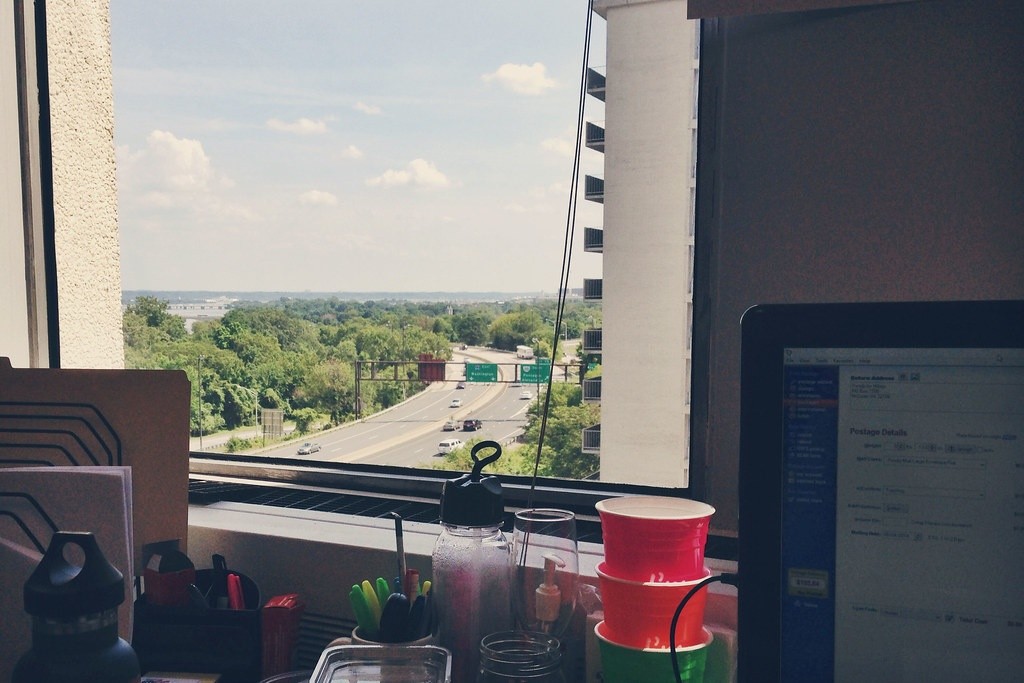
top-left (438, 438), bottom-right (465, 455)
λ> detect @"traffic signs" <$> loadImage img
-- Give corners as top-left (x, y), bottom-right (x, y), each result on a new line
top-left (521, 365), bottom-right (550, 383)
top-left (466, 363), bottom-right (497, 382)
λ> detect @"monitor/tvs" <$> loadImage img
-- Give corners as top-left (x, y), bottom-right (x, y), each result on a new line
top-left (733, 299), bottom-right (1024, 683)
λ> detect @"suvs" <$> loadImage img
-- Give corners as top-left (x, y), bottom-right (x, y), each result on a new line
top-left (463, 419), bottom-right (482, 431)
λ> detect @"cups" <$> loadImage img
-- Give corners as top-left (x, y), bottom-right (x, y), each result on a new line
top-left (593, 620), bottom-right (715, 683)
top-left (595, 562), bottom-right (711, 649)
top-left (477, 630), bottom-right (565, 683)
top-left (509, 508), bottom-right (578, 652)
top-left (325, 625), bottom-right (432, 649)
top-left (594, 495), bottom-right (716, 582)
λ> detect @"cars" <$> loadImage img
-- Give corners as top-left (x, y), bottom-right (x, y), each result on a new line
top-left (443, 420), bottom-right (461, 431)
top-left (298, 442), bottom-right (321, 454)
top-left (451, 399), bottom-right (463, 407)
top-left (459, 345), bottom-right (468, 350)
top-left (456, 382), bottom-right (467, 389)
top-left (521, 390), bottom-right (533, 399)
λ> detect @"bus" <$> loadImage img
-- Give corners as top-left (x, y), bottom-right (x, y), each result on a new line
top-left (516, 344), bottom-right (534, 359)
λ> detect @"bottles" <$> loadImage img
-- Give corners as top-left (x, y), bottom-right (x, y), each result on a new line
top-left (11, 530), bottom-right (140, 683)
top-left (432, 441), bottom-right (509, 683)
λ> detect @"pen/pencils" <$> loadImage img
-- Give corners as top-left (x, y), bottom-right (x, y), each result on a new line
top-left (213, 552), bottom-right (246, 609)
top-left (350, 514), bottom-right (431, 641)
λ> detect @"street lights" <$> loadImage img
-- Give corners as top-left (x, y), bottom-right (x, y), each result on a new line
top-left (198, 355), bottom-right (205, 450)
top-left (402, 325), bottom-right (413, 400)
top-left (558, 320), bottom-right (567, 356)
top-left (532, 338), bottom-right (540, 418)
top-left (546, 319), bottom-right (556, 345)
top-left (530, 309), bottom-right (533, 343)
